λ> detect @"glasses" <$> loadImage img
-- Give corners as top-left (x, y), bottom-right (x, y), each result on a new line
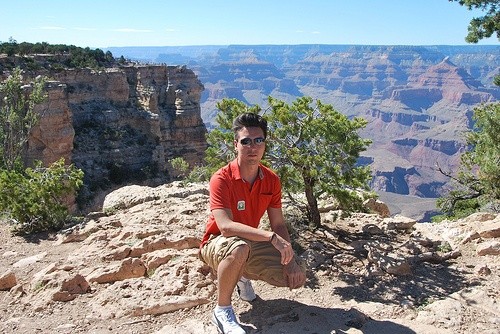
top-left (241, 137), bottom-right (264, 146)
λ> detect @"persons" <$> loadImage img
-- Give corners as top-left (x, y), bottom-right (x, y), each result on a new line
top-left (198, 112), bottom-right (307, 334)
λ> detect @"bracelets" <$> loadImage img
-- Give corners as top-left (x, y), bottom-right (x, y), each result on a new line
top-left (270, 232), bottom-right (276, 243)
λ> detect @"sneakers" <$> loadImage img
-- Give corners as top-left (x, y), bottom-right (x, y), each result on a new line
top-left (237, 279), bottom-right (256, 301)
top-left (211, 307), bottom-right (246, 334)
